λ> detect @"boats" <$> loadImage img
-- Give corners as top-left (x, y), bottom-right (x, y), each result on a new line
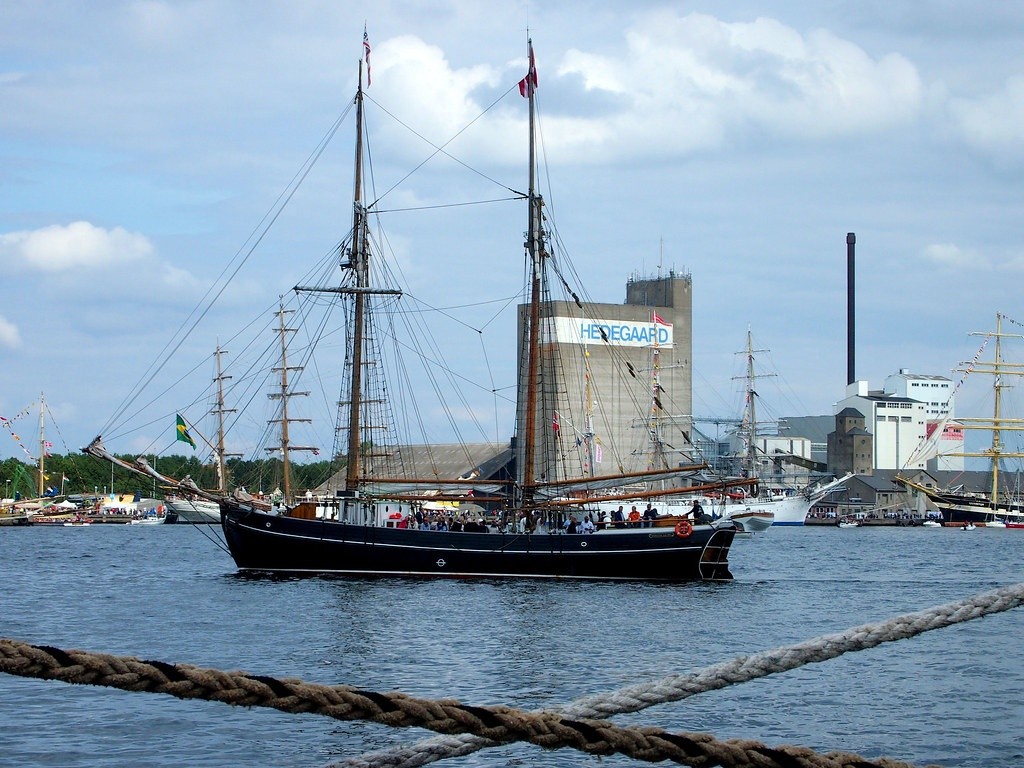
top-left (548, 473), bottom-right (857, 527)
top-left (0, 482), bottom-right (284, 530)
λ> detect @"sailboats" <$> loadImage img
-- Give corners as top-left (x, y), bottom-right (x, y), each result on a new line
top-left (79, 18), bottom-right (753, 582)
top-left (895, 311), bottom-right (1024, 526)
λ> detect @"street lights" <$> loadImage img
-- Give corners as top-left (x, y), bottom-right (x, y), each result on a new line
top-left (95, 486), bottom-right (97, 494)
top-left (4, 480), bottom-right (12, 498)
top-left (103, 486), bottom-right (107, 495)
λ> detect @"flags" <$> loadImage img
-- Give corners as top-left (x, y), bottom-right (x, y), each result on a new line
top-left (362, 25), bottom-right (372, 87)
top-left (176, 414), bottom-right (196, 449)
top-left (553, 411), bottom-right (560, 431)
top-left (654, 315), bottom-right (670, 326)
top-left (0, 395), bottom-right (54, 481)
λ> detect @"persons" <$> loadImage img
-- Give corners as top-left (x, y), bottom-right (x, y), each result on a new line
top-left (8, 496), bottom-right (166, 518)
top-left (407, 501), bottom-right (660, 535)
top-left (686, 500), bottom-right (705, 524)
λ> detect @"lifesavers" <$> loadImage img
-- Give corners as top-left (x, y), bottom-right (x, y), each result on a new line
top-left (674, 521), bottom-right (693, 538)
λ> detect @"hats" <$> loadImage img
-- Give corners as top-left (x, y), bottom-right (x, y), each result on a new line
top-left (585, 516), bottom-right (589, 519)
top-left (424, 518), bottom-right (429, 521)
top-left (693, 500), bottom-right (698, 503)
